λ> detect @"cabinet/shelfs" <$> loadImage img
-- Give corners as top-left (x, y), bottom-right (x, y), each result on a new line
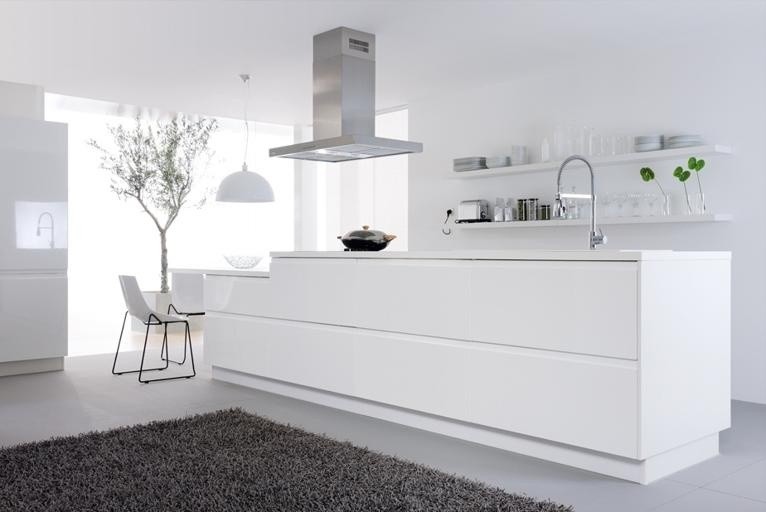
top-left (448, 142), bottom-right (736, 233)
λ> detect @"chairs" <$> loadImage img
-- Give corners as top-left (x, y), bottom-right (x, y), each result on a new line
top-left (160, 265), bottom-right (205, 366)
top-left (108, 274), bottom-right (196, 384)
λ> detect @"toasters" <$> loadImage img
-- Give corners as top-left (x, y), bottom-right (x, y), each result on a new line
top-left (456, 199), bottom-right (492, 224)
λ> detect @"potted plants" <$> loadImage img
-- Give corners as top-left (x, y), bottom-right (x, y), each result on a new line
top-left (670, 166), bottom-right (695, 217)
top-left (637, 165), bottom-right (674, 219)
top-left (83, 98), bottom-right (221, 337)
top-left (686, 155), bottom-right (711, 216)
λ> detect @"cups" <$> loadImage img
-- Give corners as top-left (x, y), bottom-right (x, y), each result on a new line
top-left (509, 145), bottom-right (529, 164)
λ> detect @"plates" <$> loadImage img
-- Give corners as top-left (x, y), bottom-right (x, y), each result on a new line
top-left (453, 155), bottom-right (511, 173)
top-left (633, 134), bottom-right (705, 152)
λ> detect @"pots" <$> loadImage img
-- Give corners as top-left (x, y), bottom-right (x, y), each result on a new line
top-left (335, 224), bottom-right (397, 250)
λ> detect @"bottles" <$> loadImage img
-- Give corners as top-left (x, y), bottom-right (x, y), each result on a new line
top-left (494, 196), bottom-right (551, 221)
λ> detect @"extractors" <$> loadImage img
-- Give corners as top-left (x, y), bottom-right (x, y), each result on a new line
top-left (268, 25), bottom-right (424, 165)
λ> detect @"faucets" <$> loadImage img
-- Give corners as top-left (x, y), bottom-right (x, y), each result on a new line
top-left (556, 157), bottom-right (606, 248)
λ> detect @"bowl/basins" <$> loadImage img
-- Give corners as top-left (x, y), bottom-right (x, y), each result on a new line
top-left (224, 252), bottom-right (265, 268)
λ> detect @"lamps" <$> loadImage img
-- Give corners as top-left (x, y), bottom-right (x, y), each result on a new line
top-left (213, 72), bottom-right (277, 204)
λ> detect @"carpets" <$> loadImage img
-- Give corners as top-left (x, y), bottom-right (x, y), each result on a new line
top-left (1, 404), bottom-right (577, 512)
